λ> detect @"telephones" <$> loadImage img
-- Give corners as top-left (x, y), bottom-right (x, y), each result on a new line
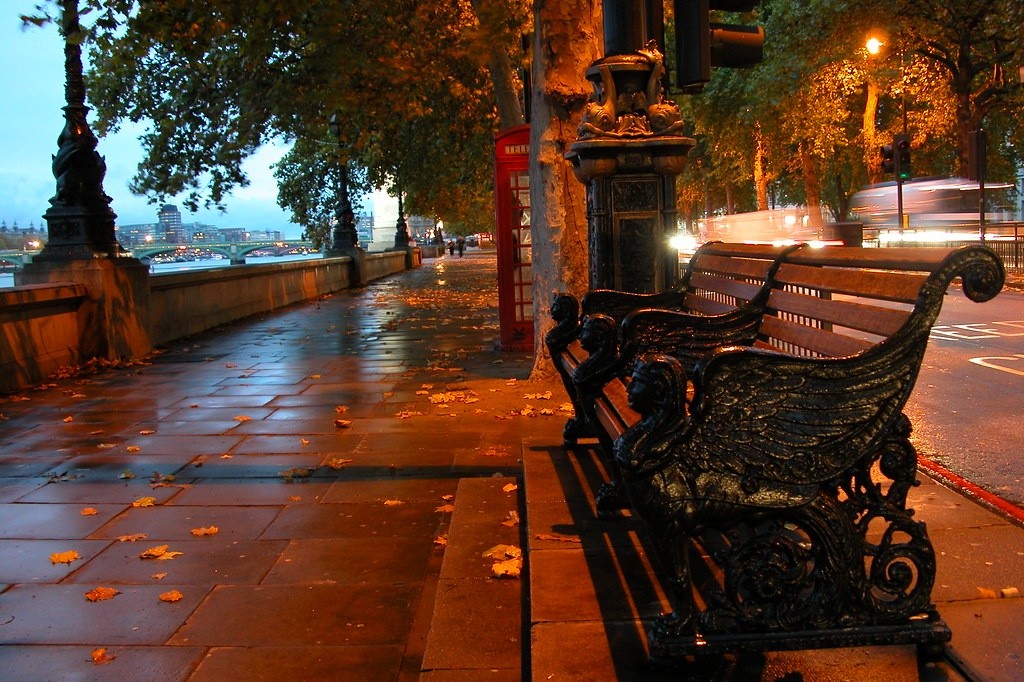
top-left (513, 193), bottom-right (525, 230)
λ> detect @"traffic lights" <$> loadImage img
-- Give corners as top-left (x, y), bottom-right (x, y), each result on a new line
top-left (880, 145), bottom-right (894, 173)
top-left (898, 140), bottom-right (910, 179)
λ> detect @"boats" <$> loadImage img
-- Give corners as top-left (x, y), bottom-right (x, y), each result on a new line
top-left (302, 251), bottom-right (307, 256)
top-left (211, 254), bottom-right (222, 261)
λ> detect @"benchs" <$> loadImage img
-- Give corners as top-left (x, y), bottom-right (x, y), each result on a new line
top-left (545, 241), bottom-right (1006, 682)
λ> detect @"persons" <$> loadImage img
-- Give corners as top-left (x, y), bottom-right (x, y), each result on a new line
top-left (448, 239), bottom-right (456, 256)
top-left (456, 235), bottom-right (465, 258)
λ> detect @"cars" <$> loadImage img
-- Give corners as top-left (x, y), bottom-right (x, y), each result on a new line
top-left (414, 238), bottom-right (426, 246)
top-left (696, 231), bottom-right (720, 243)
top-left (465, 236), bottom-right (478, 247)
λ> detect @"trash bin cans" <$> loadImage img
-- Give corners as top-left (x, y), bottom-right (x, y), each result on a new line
top-left (560, 39), bottom-right (699, 294)
